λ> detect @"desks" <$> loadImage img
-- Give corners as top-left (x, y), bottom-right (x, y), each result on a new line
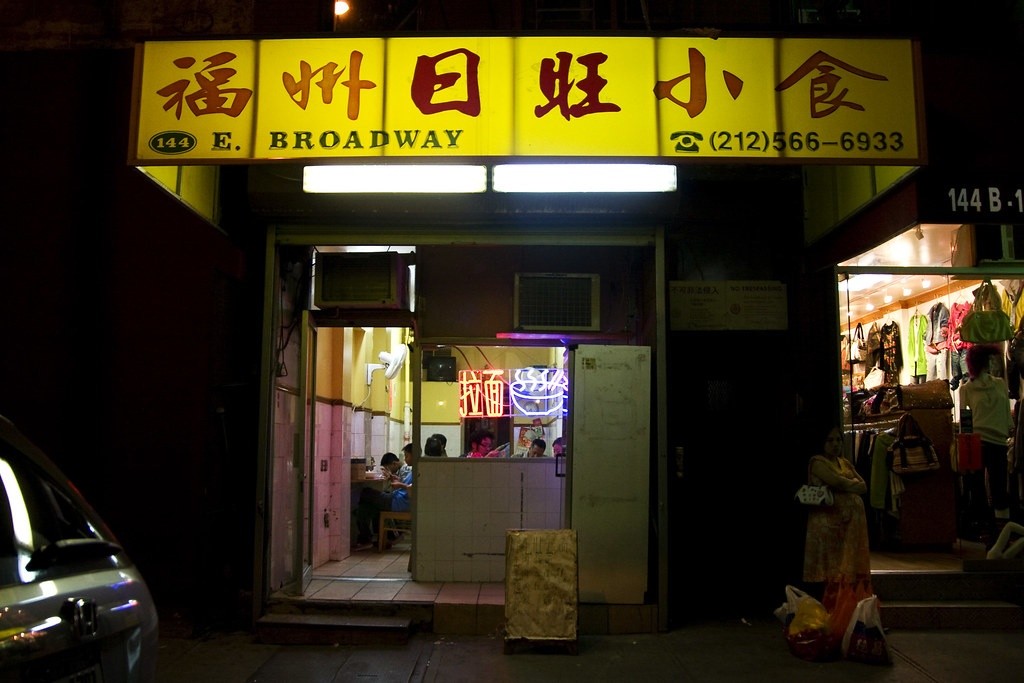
top-left (350, 476), bottom-right (389, 544)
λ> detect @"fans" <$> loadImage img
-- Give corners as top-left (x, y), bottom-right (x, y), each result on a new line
top-left (367, 344), bottom-right (407, 386)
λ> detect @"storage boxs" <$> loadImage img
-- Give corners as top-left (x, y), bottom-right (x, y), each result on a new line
top-left (350, 458), bottom-right (367, 479)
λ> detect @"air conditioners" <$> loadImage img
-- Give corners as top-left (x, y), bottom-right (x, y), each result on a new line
top-left (314, 252), bottom-right (410, 311)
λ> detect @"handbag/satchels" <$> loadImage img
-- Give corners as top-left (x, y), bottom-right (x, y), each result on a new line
top-left (885, 415), bottom-right (941, 474)
top-left (955, 280), bottom-right (1015, 342)
top-left (783, 574), bottom-right (891, 667)
top-left (794, 456), bottom-right (835, 507)
top-left (846, 323), bottom-right (867, 363)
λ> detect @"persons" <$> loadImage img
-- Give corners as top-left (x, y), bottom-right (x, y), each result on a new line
top-left (553, 437), bottom-right (563, 456)
top-left (354, 433), bottom-right (448, 551)
top-left (987, 522), bottom-right (1024, 560)
top-left (509, 439), bottom-right (547, 457)
top-left (801, 422), bottom-right (873, 601)
top-left (459, 430), bottom-right (495, 457)
top-left (958, 345), bottom-right (1011, 520)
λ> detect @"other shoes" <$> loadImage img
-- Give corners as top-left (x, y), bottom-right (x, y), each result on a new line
top-left (352, 542), bottom-right (372, 551)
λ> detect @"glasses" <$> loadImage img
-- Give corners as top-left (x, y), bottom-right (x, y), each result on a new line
top-left (478, 442), bottom-right (492, 449)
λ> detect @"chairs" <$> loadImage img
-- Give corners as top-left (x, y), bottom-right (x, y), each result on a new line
top-left (379, 483), bottom-right (415, 553)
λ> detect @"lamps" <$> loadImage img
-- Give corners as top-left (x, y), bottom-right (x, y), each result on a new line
top-left (914, 223), bottom-right (925, 240)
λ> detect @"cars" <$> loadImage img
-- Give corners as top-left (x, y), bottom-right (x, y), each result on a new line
top-left (0, 415), bottom-right (159, 683)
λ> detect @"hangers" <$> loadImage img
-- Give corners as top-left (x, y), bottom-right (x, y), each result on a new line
top-left (886, 314), bottom-right (892, 323)
top-left (955, 286), bottom-right (966, 303)
top-left (913, 306), bottom-right (922, 317)
top-left (844, 419), bottom-right (899, 435)
